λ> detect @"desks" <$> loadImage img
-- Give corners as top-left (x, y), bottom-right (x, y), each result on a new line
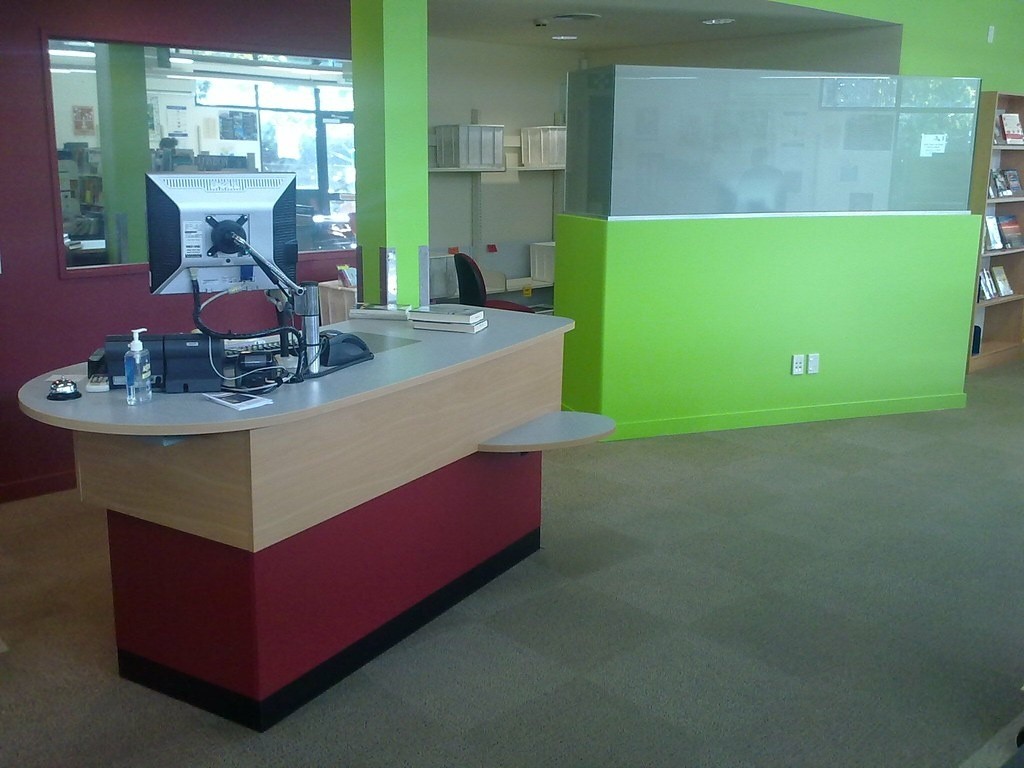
top-left (64, 237), bottom-right (106, 267)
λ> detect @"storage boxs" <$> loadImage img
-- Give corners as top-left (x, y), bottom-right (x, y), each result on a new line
top-left (429, 252), bottom-right (460, 298)
top-left (527, 304), bottom-right (554, 316)
top-left (435, 124), bottom-right (505, 168)
top-left (521, 126), bottom-right (567, 167)
top-left (318, 280), bottom-right (358, 327)
top-left (529, 241), bottom-right (555, 282)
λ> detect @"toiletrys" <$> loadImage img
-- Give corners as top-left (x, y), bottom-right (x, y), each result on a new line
top-left (124, 328), bottom-right (153, 406)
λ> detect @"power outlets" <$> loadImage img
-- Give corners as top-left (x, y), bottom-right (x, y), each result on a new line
top-left (792, 355), bottom-right (804, 375)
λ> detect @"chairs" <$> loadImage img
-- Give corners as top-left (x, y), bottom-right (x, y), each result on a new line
top-left (454, 254), bottom-right (534, 314)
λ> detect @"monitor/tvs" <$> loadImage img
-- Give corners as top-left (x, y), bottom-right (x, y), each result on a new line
top-left (144, 171), bottom-right (299, 295)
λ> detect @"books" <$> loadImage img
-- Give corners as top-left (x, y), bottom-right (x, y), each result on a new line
top-left (984, 215), bottom-right (1024, 251)
top-left (991, 110), bottom-right (1024, 146)
top-left (979, 264), bottom-right (1013, 301)
top-left (988, 168), bottom-right (1023, 199)
top-left (407, 306), bottom-right (490, 335)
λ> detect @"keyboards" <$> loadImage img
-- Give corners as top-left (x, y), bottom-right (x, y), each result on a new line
top-left (235, 339), bottom-right (301, 363)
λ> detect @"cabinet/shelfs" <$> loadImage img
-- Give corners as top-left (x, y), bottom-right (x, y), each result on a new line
top-left (428, 109), bottom-right (567, 315)
top-left (966, 91), bottom-right (1024, 376)
top-left (77, 148), bottom-right (103, 207)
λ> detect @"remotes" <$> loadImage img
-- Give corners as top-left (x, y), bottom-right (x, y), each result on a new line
top-left (86, 374), bottom-right (110, 393)
